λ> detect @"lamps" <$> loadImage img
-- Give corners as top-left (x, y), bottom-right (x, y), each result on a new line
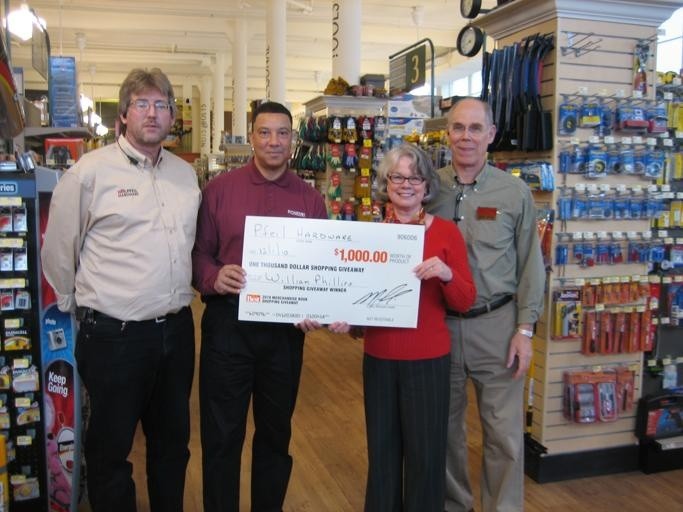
top-left (1, 1), bottom-right (109, 139)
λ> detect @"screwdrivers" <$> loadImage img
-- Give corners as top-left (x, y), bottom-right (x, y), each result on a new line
top-left (563, 154), bottom-right (569, 187)
top-left (559, 247), bottom-right (567, 276)
top-left (559, 199), bottom-right (567, 232)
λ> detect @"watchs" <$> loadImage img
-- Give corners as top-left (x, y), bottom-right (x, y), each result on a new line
top-left (518, 328), bottom-right (533, 339)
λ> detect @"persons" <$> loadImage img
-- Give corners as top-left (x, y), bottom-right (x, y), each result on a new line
top-left (421, 99), bottom-right (545, 511)
top-left (192, 101), bottom-right (327, 512)
top-left (329, 144), bottom-right (477, 512)
top-left (41, 69), bottom-right (202, 510)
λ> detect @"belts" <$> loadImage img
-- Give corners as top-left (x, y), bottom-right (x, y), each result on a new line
top-left (79, 301), bottom-right (193, 337)
top-left (443, 293), bottom-right (513, 324)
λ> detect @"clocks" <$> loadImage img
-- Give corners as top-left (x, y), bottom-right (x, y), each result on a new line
top-left (459, 0), bottom-right (482, 19)
top-left (455, 24), bottom-right (483, 56)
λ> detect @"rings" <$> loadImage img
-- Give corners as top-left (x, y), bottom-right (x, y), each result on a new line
top-left (429, 266), bottom-right (433, 271)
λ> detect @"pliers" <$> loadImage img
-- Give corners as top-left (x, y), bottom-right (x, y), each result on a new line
top-left (635, 57), bottom-right (646, 94)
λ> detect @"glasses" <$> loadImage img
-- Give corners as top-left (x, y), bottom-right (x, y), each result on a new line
top-left (386, 172), bottom-right (427, 186)
top-left (124, 98), bottom-right (175, 113)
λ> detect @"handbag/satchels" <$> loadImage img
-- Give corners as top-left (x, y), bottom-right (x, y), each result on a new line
top-left (74, 305), bottom-right (96, 327)
